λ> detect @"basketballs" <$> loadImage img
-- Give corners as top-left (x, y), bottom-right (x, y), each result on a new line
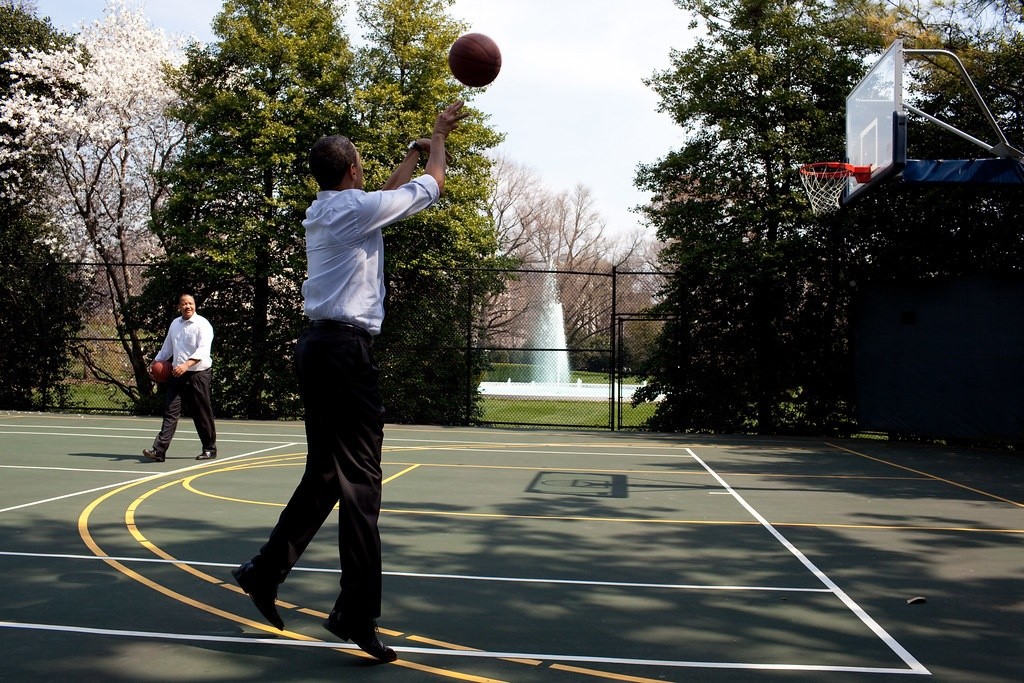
top-left (449, 32), bottom-right (502, 88)
top-left (151, 360), bottom-right (173, 383)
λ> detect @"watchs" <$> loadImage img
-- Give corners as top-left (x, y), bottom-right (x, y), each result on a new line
top-left (407, 141), bottom-right (423, 153)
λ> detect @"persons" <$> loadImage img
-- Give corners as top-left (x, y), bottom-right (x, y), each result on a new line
top-left (143, 293), bottom-right (218, 463)
top-left (230, 100), bottom-right (470, 661)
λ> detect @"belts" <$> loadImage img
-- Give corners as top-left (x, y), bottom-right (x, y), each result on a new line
top-left (307, 320), bottom-right (375, 348)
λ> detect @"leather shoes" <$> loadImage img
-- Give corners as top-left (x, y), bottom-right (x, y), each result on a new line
top-left (322, 605), bottom-right (397, 660)
top-left (196, 450), bottom-right (217, 459)
top-left (230, 559), bottom-right (284, 631)
top-left (143, 448), bottom-right (165, 462)
